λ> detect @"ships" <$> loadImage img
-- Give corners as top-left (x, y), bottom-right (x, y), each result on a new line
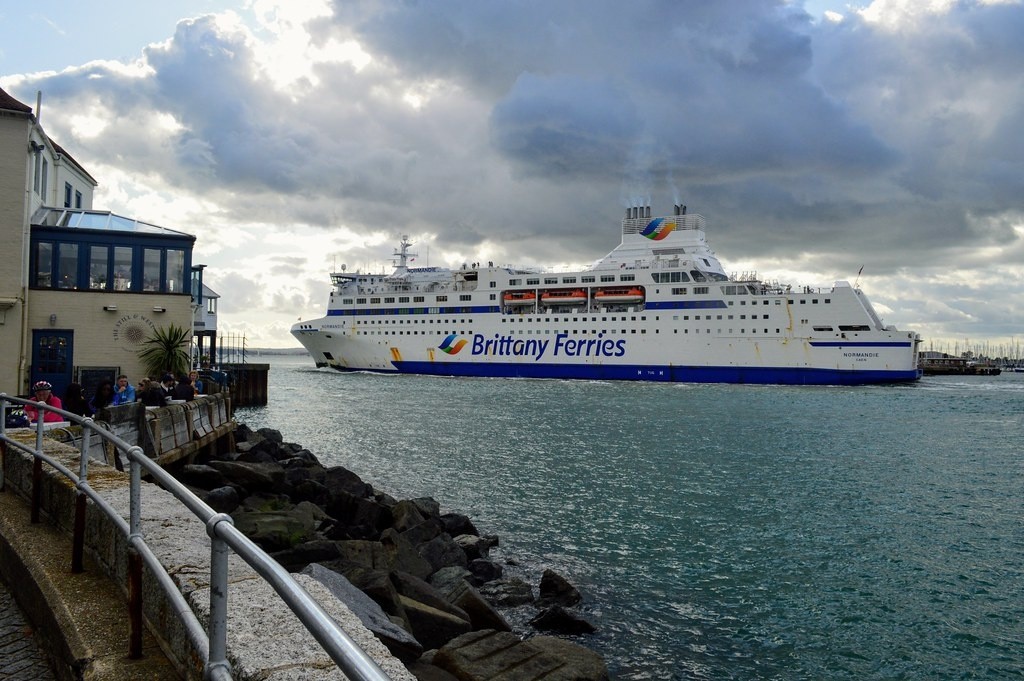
top-left (290, 203), bottom-right (920, 385)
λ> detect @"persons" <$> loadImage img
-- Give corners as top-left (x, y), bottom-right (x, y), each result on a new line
top-left (13, 368), bottom-right (202, 423)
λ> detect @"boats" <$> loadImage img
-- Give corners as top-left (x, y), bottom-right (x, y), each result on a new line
top-left (595, 288), bottom-right (644, 304)
top-left (504, 292), bottom-right (537, 306)
top-left (540, 290), bottom-right (588, 305)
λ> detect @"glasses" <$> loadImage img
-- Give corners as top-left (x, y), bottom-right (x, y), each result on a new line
top-left (139, 383), bottom-right (145, 387)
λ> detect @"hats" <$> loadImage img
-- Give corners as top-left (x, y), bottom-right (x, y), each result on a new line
top-left (32, 381), bottom-right (53, 392)
top-left (163, 374), bottom-right (174, 381)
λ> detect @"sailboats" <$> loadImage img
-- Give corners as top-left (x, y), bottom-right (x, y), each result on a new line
top-left (917, 335), bottom-right (1024, 376)
